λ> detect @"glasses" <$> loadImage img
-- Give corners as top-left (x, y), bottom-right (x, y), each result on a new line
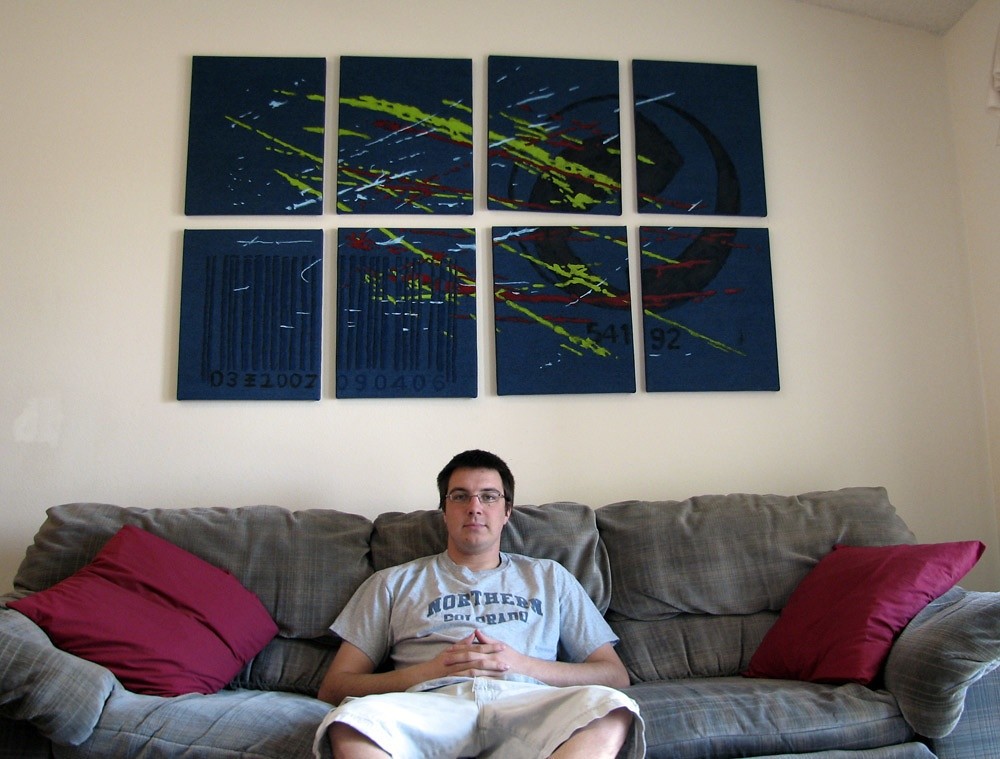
top-left (445, 491), bottom-right (504, 503)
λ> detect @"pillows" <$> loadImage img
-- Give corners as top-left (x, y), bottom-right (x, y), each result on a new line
top-left (742, 540), bottom-right (987, 688)
top-left (5, 523), bottom-right (279, 697)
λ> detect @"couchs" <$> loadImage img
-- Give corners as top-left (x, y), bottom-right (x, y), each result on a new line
top-left (0, 487), bottom-right (1000, 759)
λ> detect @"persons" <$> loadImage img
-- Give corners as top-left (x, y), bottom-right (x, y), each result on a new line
top-left (313, 449), bottom-right (648, 759)
